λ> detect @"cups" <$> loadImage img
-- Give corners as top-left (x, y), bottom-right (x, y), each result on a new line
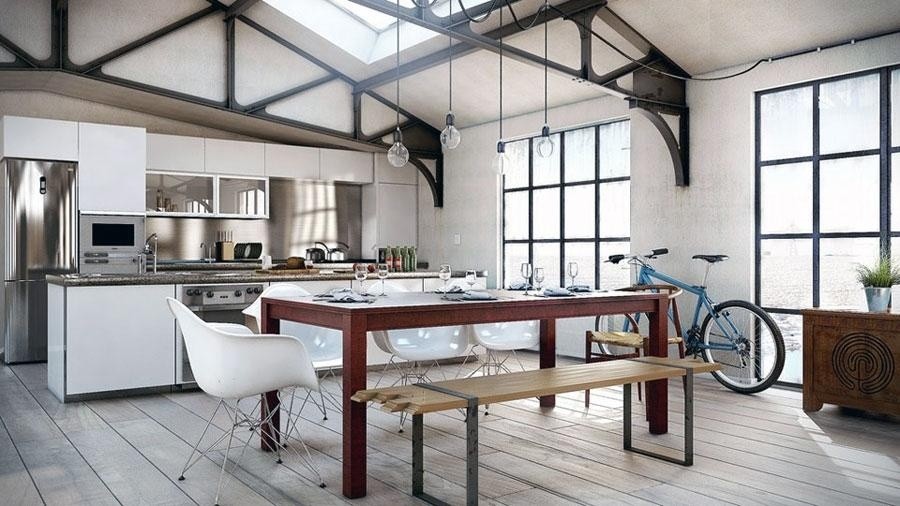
top-left (261, 255), bottom-right (272, 271)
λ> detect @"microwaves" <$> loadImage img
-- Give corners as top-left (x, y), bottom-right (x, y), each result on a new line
top-left (79, 216), bottom-right (147, 275)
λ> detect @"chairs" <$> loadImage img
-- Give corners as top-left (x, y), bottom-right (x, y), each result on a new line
top-left (581, 281), bottom-right (683, 409)
top-left (454, 320), bottom-right (541, 416)
top-left (165, 294), bottom-right (326, 505)
top-left (241, 282), bottom-right (346, 429)
top-left (365, 279), bottom-right (468, 435)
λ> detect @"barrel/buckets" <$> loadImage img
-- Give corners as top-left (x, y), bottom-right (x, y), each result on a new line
top-left (860, 285), bottom-right (892, 312)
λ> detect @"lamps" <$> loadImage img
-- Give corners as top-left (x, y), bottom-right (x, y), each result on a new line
top-left (387, 0), bottom-right (555, 175)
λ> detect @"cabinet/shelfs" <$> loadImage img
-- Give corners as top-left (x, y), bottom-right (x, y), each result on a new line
top-left (350, 280), bottom-right (424, 372)
top-left (424, 277), bottom-right (487, 367)
top-left (145, 169), bottom-right (271, 221)
top-left (44, 275), bottom-right (175, 403)
top-left (270, 280), bottom-right (351, 378)
top-left (800, 309), bottom-right (899, 419)
top-left (2, 115), bottom-right (79, 163)
top-left (146, 132), bottom-right (374, 183)
top-left (79, 121), bottom-right (147, 217)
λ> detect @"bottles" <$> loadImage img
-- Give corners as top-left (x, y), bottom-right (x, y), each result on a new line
top-left (381, 245), bottom-right (417, 272)
top-left (156, 188), bottom-right (177, 211)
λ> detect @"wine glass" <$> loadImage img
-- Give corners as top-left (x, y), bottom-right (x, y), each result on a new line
top-left (355, 262), bottom-right (368, 297)
top-left (521, 263), bottom-right (544, 297)
top-left (439, 265), bottom-right (477, 299)
top-left (377, 264), bottom-right (388, 296)
top-left (567, 262), bottom-right (578, 295)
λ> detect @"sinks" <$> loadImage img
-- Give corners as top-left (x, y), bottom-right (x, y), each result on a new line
top-left (180, 258), bottom-right (211, 264)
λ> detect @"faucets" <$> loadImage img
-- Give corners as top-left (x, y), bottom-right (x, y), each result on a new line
top-left (199, 241), bottom-right (209, 258)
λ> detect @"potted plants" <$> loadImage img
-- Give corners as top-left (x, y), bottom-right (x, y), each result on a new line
top-left (856, 253), bottom-right (900, 313)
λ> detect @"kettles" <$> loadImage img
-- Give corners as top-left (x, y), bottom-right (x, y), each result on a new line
top-left (306, 241), bottom-right (349, 262)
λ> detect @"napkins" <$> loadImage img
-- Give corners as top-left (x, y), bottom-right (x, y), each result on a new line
top-left (314, 284), bottom-right (352, 301)
top-left (568, 284), bottom-right (591, 293)
top-left (435, 283), bottom-right (494, 303)
top-left (510, 282), bottom-right (535, 292)
top-left (328, 291), bottom-right (378, 304)
top-left (544, 286), bottom-right (573, 297)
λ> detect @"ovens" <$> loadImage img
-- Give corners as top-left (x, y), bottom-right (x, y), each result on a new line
top-left (176, 284), bottom-right (263, 385)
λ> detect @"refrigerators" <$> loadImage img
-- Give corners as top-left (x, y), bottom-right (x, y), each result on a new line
top-left (0, 157), bottom-right (79, 364)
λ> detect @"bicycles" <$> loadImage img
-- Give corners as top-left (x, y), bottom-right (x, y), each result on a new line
top-left (595, 249), bottom-right (785, 394)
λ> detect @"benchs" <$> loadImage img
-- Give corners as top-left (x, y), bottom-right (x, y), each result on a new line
top-left (351, 356), bottom-right (722, 505)
